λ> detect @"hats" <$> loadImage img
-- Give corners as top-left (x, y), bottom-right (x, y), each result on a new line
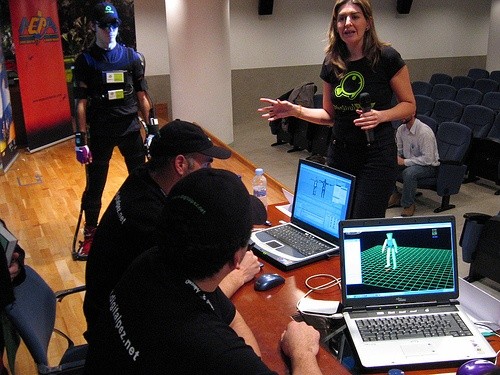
top-left (93, 2), bottom-right (118, 23)
top-left (149, 119), bottom-right (231, 165)
top-left (164, 168), bottom-right (267, 245)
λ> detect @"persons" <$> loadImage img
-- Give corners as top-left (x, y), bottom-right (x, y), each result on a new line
top-left (388, 105), bottom-right (439, 217)
top-left (88, 169), bottom-right (322, 375)
top-left (82, 119), bottom-right (231, 343)
top-left (257, 0), bottom-right (416, 220)
top-left (0, 217), bottom-right (25, 375)
top-left (64, 2), bottom-right (161, 256)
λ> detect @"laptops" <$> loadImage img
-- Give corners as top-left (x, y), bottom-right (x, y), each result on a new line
top-left (244, 159), bottom-right (357, 272)
top-left (339, 213), bottom-right (497, 375)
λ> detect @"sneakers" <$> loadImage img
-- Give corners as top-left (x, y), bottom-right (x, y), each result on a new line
top-left (78, 222), bottom-right (99, 257)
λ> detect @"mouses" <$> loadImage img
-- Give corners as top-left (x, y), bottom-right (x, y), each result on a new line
top-left (456, 359), bottom-right (499, 375)
top-left (254, 274), bottom-right (286, 291)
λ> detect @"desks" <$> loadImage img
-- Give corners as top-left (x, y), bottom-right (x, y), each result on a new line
top-left (231, 201), bottom-right (500, 375)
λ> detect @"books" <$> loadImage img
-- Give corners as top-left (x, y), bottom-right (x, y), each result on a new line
top-left (0, 220), bottom-right (18, 268)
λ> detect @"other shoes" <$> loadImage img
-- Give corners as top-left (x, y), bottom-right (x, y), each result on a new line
top-left (401, 203), bottom-right (415, 216)
top-left (388, 192), bottom-right (402, 207)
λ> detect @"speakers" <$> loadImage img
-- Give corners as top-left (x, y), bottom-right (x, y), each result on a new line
top-left (258, 0), bottom-right (274, 15)
top-left (397, 0), bottom-right (413, 14)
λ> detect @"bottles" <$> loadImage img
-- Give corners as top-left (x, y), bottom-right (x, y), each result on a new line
top-left (251, 168), bottom-right (267, 211)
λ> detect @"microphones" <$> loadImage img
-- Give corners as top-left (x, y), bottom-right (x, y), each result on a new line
top-left (360, 92), bottom-right (375, 142)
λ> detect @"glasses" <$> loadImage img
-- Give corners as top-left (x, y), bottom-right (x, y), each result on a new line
top-left (94, 21), bottom-right (118, 30)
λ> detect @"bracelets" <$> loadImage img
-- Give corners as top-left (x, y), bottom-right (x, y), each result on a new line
top-left (297, 105), bottom-right (302, 118)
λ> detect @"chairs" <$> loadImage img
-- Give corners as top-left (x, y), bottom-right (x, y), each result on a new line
top-left (3, 264), bottom-right (90, 375)
top-left (271, 69), bottom-right (500, 285)
